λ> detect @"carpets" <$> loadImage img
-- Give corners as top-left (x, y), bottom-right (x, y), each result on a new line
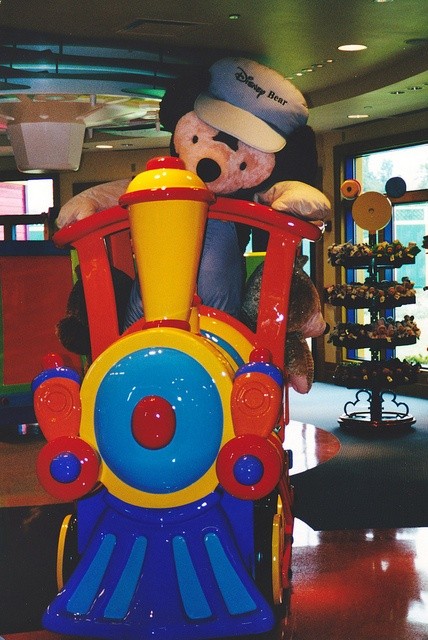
top-left (275, 381), bottom-right (428, 530)
